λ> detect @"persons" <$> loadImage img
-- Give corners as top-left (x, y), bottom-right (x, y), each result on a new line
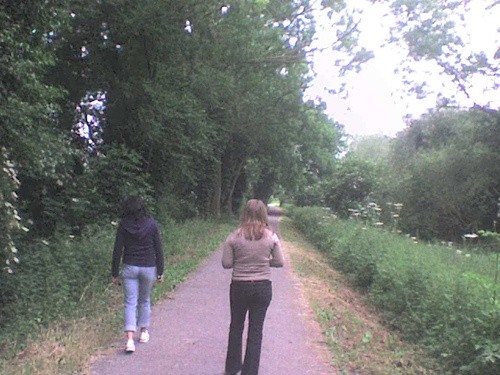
top-left (222, 200), bottom-right (284, 375)
top-left (112, 196), bottom-right (164, 352)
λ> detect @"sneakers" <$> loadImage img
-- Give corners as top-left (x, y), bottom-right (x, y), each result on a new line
top-left (139, 330), bottom-right (149, 343)
top-left (125, 342), bottom-right (135, 352)
